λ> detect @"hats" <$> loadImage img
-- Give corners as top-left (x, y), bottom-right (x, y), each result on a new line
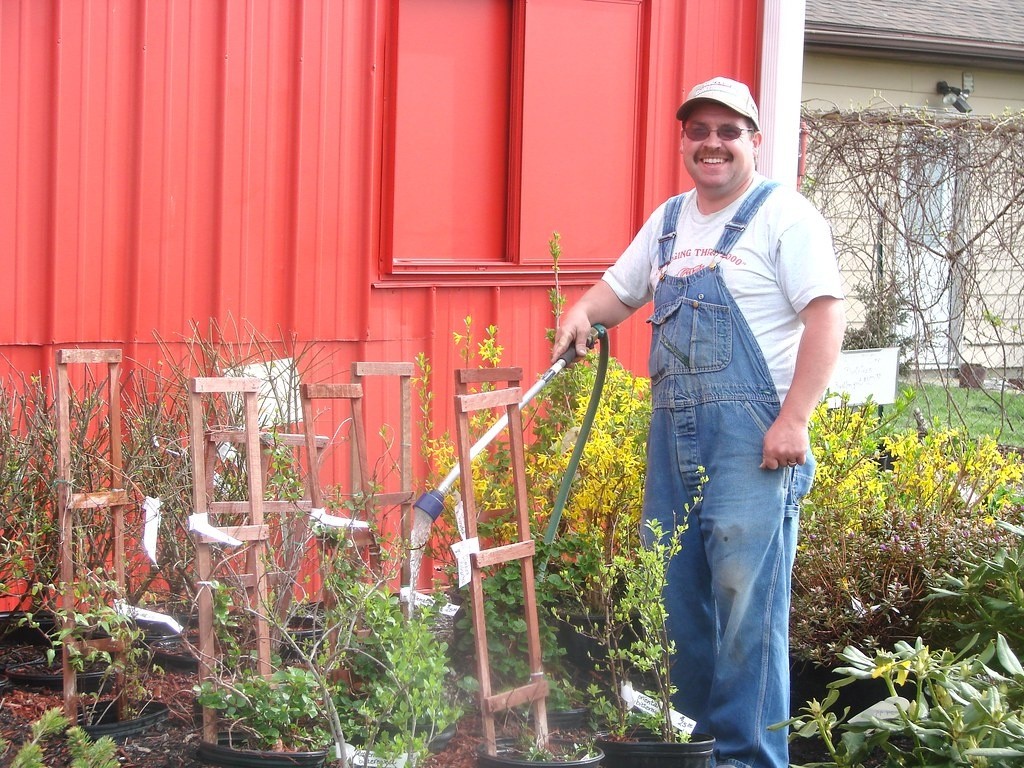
top-left (676, 76), bottom-right (760, 131)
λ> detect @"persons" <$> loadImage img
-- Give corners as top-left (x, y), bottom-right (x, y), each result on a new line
top-left (550, 76), bottom-right (846, 768)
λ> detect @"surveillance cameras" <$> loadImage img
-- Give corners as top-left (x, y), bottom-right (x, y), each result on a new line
top-left (953, 98), bottom-right (973, 113)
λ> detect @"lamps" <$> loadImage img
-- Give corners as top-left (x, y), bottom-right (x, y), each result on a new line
top-left (937, 80), bottom-right (973, 115)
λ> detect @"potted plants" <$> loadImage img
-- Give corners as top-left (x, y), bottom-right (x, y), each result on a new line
top-left (0, 228), bottom-right (1024, 768)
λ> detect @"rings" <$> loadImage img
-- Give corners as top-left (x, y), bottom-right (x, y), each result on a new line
top-left (788, 461), bottom-right (797, 466)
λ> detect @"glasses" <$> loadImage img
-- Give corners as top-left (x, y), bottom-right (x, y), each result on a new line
top-left (681, 124), bottom-right (754, 141)
